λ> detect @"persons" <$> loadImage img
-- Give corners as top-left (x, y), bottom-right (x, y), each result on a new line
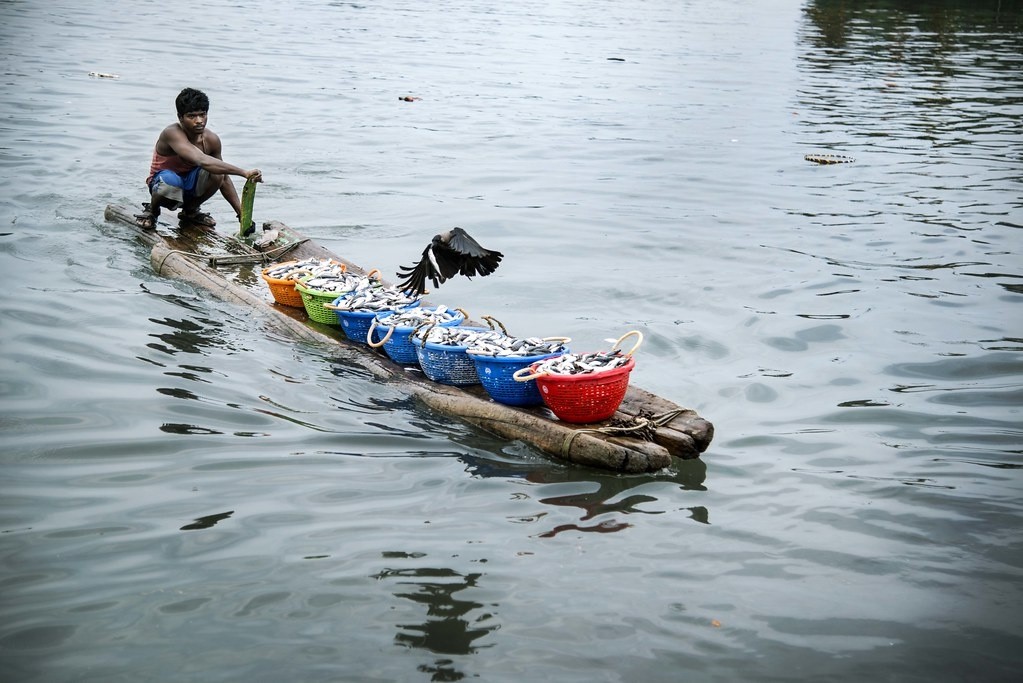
top-left (134, 88), bottom-right (263, 229)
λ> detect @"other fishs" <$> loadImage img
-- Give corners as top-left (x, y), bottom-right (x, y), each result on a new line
top-left (263, 256), bottom-right (631, 376)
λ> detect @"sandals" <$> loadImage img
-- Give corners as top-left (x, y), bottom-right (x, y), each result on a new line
top-left (178, 208), bottom-right (217, 227)
top-left (133, 203), bottom-right (161, 228)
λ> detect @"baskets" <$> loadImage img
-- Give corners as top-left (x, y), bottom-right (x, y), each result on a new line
top-left (323, 286), bottom-right (430, 343)
top-left (465, 336), bottom-right (573, 406)
top-left (261, 257), bottom-right (346, 308)
top-left (513, 330), bottom-right (643, 424)
top-left (295, 269), bottom-right (381, 324)
top-left (410, 315), bottom-right (509, 385)
top-left (366, 308), bottom-right (469, 363)
